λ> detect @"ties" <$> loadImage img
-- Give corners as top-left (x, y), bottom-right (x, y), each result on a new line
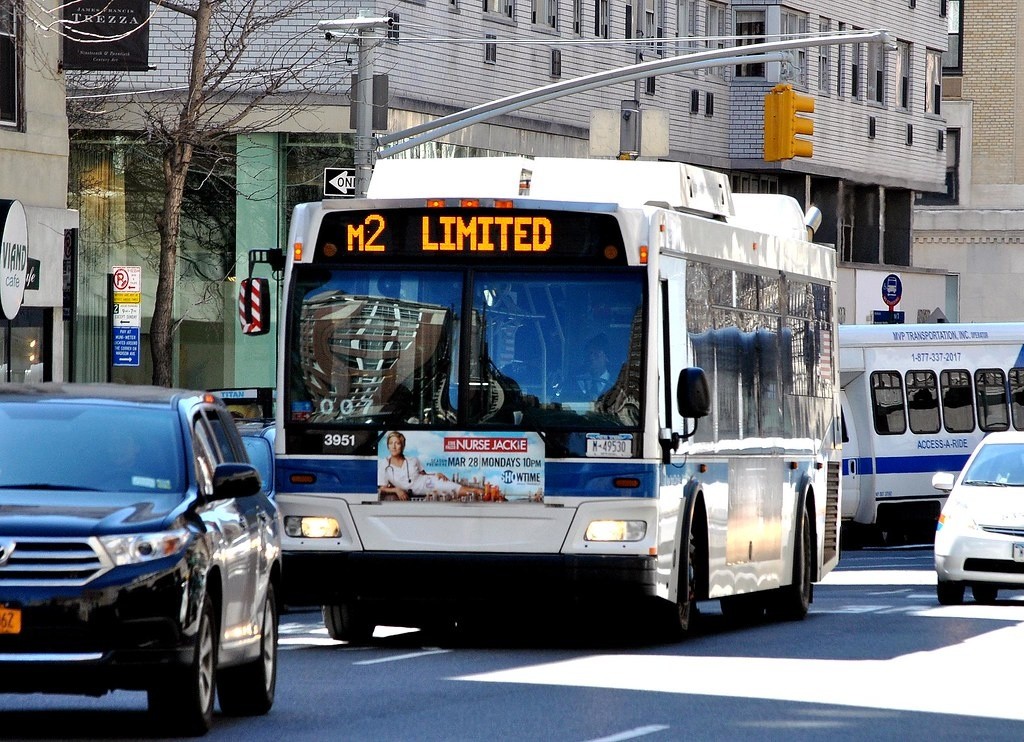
top-left (591, 380), bottom-right (598, 395)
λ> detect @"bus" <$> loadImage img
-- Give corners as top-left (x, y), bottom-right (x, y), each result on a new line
top-left (833, 322), bottom-right (1024, 549)
top-left (242, 156), bottom-right (842, 638)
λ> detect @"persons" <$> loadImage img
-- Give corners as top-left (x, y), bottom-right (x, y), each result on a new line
top-left (378, 431), bottom-right (485, 501)
top-left (566, 332), bottom-right (620, 403)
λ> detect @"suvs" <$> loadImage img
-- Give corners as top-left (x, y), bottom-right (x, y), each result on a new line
top-left (0, 383), bottom-right (287, 740)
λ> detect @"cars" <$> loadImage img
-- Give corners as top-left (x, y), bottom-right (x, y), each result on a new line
top-left (225, 417), bottom-right (280, 508)
top-left (930, 428), bottom-right (1023, 605)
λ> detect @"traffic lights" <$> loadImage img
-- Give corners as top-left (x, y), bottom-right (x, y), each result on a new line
top-left (763, 91), bottom-right (815, 161)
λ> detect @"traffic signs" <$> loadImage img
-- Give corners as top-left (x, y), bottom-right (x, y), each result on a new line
top-left (325, 167), bottom-right (373, 197)
